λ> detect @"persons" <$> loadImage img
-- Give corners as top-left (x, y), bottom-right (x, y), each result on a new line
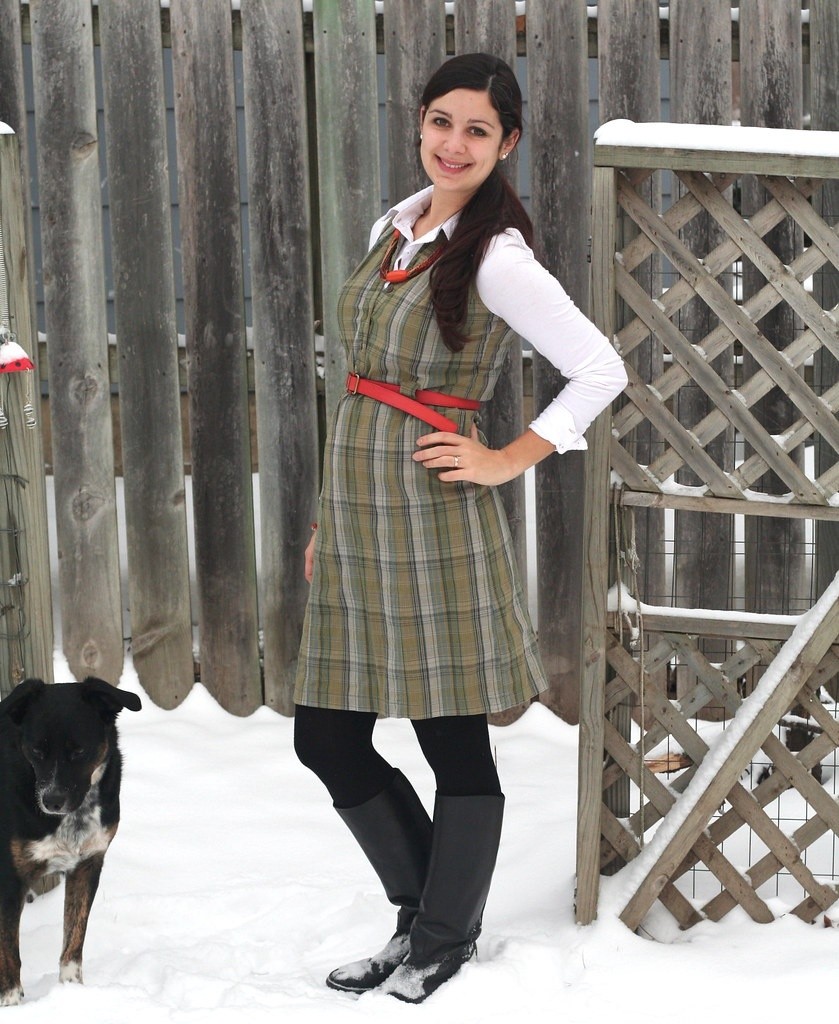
top-left (293, 51), bottom-right (627, 1003)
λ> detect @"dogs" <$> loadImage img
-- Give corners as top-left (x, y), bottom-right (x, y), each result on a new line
top-left (0, 675), bottom-right (142, 1007)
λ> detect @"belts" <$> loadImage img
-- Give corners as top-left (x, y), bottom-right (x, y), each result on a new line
top-left (344, 372), bottom-right (483, 433)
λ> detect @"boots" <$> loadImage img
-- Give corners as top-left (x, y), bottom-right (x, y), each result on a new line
top-left (358, 789), bottom-right (506, 1004)
top-left (326, 769), bottom-right (434, 995)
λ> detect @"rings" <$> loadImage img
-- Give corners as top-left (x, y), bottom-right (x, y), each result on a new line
top-left (455, 457), bottom-right (458, 466)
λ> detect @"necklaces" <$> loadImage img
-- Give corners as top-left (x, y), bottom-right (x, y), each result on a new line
top-left (379, 229), bottom-right (446, 282)
